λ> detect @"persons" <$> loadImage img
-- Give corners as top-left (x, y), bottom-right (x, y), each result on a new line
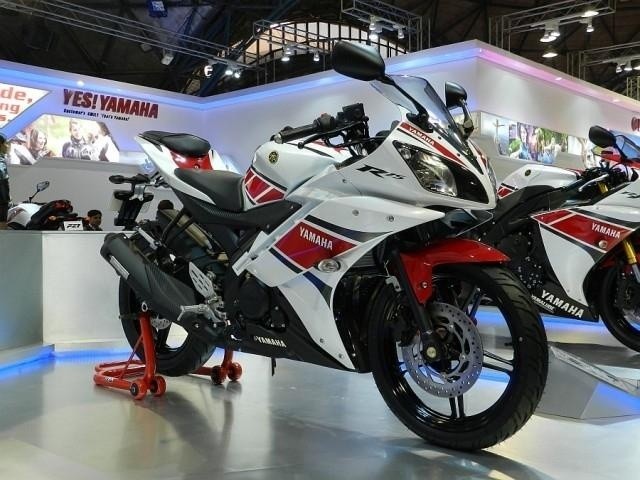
top-left (83, 210), bottom-right (103, 231)
top-left (0, 132), bottom-right (11, 222)
top-left (19, 118), bottom-right (117, 162)
top-left (511, 123), bottom-right (561, 163)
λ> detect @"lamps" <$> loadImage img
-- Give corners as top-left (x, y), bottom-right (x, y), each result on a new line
top-left (530, 3), bottom-right (640, 74)
top-left (161, 14), bottom-right (404, 82)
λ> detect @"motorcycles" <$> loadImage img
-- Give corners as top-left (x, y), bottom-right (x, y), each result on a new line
top-left (489, 123), bottom-right (640, 351)
top-left (101, 41), bottom-right (557, 454)
top-left (6, 179), bottom-right (83, 231)
